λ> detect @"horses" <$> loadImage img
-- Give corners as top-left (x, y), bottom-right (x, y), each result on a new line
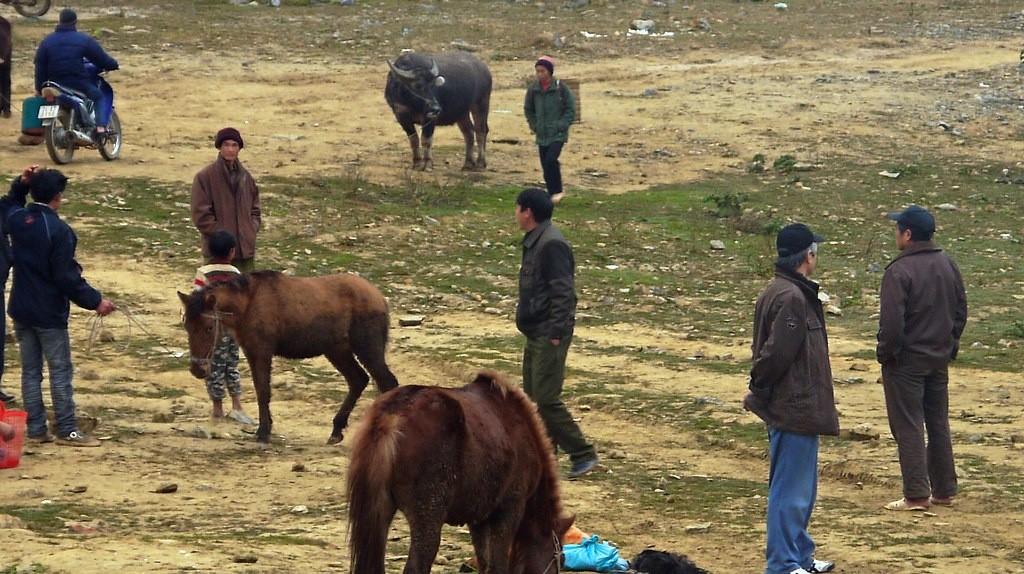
top-left (176, 269), bottom-right (398, 448)
top-left (344, 370), bottom-right (577, 574)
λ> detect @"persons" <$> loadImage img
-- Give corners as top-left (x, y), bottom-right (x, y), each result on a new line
top-left (743, 222), bottom-right (841, 574)
top-left (523, 54), bottom-right (575, 206)
top-left (33, 8), bottom-right (119, 134)
top-left (0, 164), bottom-right (119, 447)
top-left (0, 16), bottom-right (13, 119)
top-left (193, 230), bottom-right (257, 425)
top-left (190, 126), bottom-right (262, 272)
top-left (516, 190), bottom-right (597, 478)
top-left (876, 205), bottom-right (967, 511)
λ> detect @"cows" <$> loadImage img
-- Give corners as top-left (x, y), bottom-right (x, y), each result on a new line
top-left (383, 51), bottom-right (495, 170)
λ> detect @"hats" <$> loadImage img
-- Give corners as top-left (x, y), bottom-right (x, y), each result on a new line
top-left (887, 205), bottom-right (937, 235)
top-left (534, 56), bottom-right (555, 76)
top-left (214, 127), bottom-right (244, 150)
top-left (777, 222), bottom-right (824, 257)
top-left (59, 8), bottom-right (78, 25)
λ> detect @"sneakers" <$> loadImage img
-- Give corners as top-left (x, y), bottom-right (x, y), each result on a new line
top-left (787, 568), bottom-right (811, 574)
top-left (801, 558), bottom-right (835, 573)
top-left (28, 431), bottom-right (56, 443)
top-left (55, 428), bottom-right (101, 447)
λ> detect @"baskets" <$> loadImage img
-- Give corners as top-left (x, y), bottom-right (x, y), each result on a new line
top-left (0, 399), bottom-right (29, 469)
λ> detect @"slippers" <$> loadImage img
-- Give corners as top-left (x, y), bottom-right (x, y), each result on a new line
top-left (883, 497), bottom-right (927, 510)
top-left (929, 494), bottom-right (953, 507)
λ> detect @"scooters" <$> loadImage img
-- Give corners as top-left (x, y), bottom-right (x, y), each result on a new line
top-left (0, 0), bottom-right (51, 17)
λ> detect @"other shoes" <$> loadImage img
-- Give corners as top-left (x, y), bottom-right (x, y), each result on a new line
top-left (551, 191), bottom-right (564, 204)
top-left (0, 391), bottom-right (15, 402)
top-left (4, 108), bottom-right (12, 118)
top-left (569, 457), bottom-right (599, 476)
top-left (228, 409), bottom-right (258, 425)
top-left (94, 127), bottom-right (111, 138)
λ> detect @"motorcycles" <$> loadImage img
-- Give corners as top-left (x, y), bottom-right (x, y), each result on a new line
top-left (39, 59), bottom-right (122, 166)
